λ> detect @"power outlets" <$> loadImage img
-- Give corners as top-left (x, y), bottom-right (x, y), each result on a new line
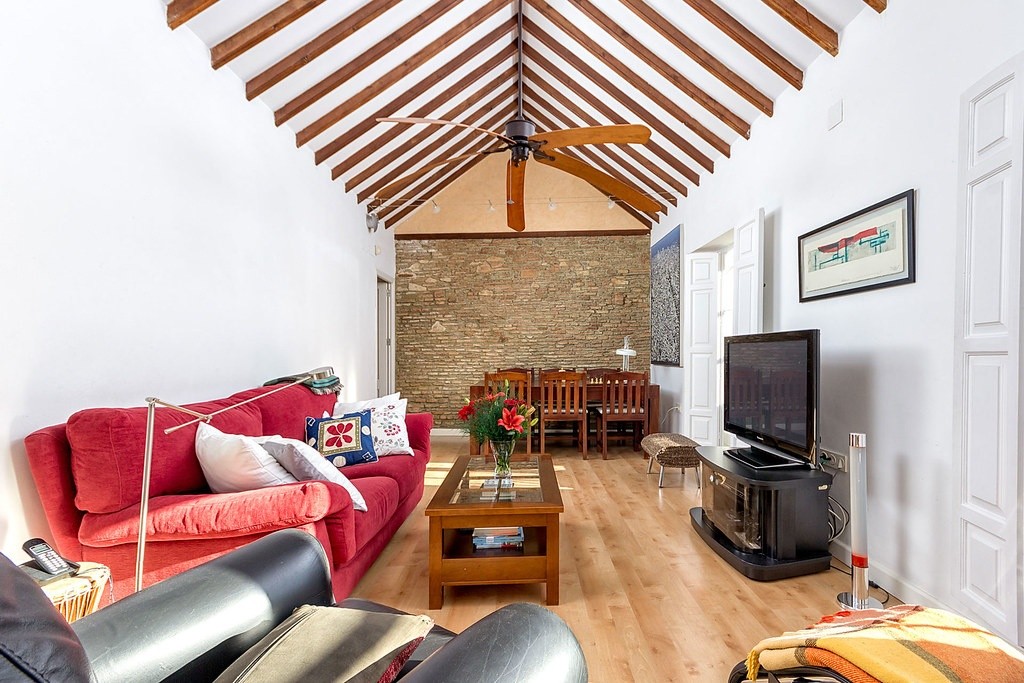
top-left (820, 448), bottom-right (847, 473)
top-left (675, 403), bottom-right (681, 413)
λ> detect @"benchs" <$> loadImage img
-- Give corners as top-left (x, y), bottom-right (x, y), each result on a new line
top-left (641, 432), bottom-right (703, 489)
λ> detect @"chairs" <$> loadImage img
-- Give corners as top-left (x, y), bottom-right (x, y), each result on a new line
top-left (483, 366), bottom-right (649, 460)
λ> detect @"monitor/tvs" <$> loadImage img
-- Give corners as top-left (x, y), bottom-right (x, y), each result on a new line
top-left (723, 329), bottom-right (821, 469)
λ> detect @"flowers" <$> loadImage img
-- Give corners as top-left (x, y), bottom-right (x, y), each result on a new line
top-left (457, 373), bottom-right (539, 446)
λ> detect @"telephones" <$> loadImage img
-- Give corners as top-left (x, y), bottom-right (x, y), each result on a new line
top-left (17, 538), bottom-right (80, 587)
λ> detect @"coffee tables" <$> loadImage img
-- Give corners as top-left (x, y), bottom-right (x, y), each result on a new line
top-left (39, 561), bottom-right (110, 624)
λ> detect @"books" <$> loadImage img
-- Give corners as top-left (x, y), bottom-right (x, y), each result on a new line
top-left (472, 527), bottom-right (524, 549)
top-left (480, 479), bottom-right (516, 501)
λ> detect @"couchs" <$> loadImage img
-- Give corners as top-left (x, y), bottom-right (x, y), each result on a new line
top-left (23, 381), bottom-right (433, 600)
top-left (0, 528), bottom-right (588, 683)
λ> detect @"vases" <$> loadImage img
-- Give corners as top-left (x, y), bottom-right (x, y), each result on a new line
top-left (490, 439), bottom-right (516, 475)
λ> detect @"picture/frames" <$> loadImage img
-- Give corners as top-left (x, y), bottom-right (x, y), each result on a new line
top-left (798, 189), bottom-right (917, 302)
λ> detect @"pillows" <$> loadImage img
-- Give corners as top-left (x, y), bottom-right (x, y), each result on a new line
top-left (265, 434), bottom-right (368, 512)
top-left (357, 398), bottom-right (415, 456)
top-left (195, 421), bottom-right (299, 494)
top-left (304, 408), bottom-right (379, 468)
top-left (212, 604), bottom-right (435, 683)
top-left (333, 391), bottom-right (400, 417)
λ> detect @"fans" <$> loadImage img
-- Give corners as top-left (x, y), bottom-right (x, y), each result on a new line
top-left (374, 0), bottom-right (662, 232)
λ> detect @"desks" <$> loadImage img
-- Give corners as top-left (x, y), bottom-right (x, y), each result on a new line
top-left (469, 379), bottom-right (660, 452)
top-left (424, 454), bottom-right (564, 611)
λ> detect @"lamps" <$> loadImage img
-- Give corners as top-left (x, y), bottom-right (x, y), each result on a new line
top-left (837, 432), bottom-right (883, 612)
